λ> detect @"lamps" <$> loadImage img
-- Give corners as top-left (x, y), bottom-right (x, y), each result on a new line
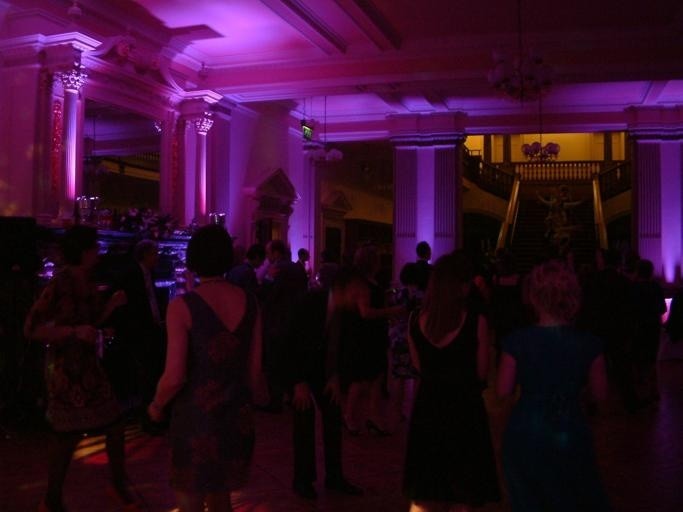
top-left (521, 95), bottom-right (560, 161)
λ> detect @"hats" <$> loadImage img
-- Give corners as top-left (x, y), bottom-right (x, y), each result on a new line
top-left (461, 230), bottom-right (495, 257)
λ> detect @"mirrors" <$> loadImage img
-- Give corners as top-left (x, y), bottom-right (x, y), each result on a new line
top-left (81, 95), bottom-right (162, 217)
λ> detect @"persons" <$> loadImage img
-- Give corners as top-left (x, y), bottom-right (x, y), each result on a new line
top-left (22, 222), bottom-right (681, 510)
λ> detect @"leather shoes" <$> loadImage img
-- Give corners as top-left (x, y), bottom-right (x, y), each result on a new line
top-left (324, 478), bottom-right (362, 495)
top-left (293, 479), bottom-right (318, 500)
